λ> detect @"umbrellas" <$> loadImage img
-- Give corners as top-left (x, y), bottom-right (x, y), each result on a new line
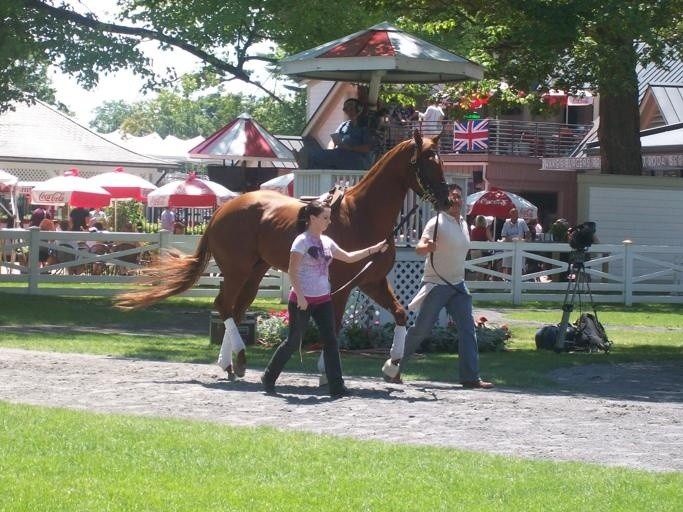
top-left (278, 19), bottom-right (487, 122)
top-left (461, 185), bottom-right (537, 270)
top-left (433, 78), bottom-right (526, 111)
top-left (25, 167), bottom-right (112, 219)
top-left (257, 172), bottom-right (295, 198)
top-left (0, 166), bottom-right (18, 196)
top-left (87, 164), bottom-right (160, 232)
top-left (188, 110), bottom-right (295, 193)
top-left (521, 74), bottom-right (594, 126)
top-left (145, 172), bottom-right (238, 233)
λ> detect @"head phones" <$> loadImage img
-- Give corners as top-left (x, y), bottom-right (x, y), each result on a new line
top-left (342, 98), bottom-right (364, 113)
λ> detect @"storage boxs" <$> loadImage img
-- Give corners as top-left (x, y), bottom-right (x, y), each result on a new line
top-left (208, 310), bottom-right (259, 345)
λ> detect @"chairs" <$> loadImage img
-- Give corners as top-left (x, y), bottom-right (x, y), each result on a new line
top-left (18, 244), bottom-right (138, 275)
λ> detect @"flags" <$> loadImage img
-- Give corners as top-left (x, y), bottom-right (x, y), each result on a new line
top-left (451, 119), bottom-right (493, 156)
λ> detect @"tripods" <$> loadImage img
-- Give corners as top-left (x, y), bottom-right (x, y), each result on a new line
top-left (557, 251), bottom-right (599, 352)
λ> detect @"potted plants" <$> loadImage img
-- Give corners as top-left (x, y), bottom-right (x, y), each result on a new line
top-left (105, 198), bottom-right (144, 232)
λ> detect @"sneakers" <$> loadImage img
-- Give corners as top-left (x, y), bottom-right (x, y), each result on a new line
top-left (261, 373), bottom-right (278, 396)
top-left (383, 371), bottom-right (403, 385)
top-left (463, 378), bottom-right (494, 389)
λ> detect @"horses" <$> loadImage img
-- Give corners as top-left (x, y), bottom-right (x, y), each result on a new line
top-left (112, 129), bottom-right (455, 387)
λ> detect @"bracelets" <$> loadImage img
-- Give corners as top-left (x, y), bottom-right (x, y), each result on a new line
top-left (368, 247), bottom-right (371, 257)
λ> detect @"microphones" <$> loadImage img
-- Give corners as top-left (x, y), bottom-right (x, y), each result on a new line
top-left (353, 111), bottom-right (361, 120)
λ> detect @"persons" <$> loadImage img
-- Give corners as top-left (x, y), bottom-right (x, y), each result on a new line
top-left (171, 221), bottom-right (186, 235)
top-left (381, 182), bottom-right (496, 391)
top-left (300, 97), bottom-right (448, 169)
top-left (9, 205), bottom-right (142, 275)
top-left (257, 198), bottom-right (391, 401)
top-left (160, 206), bottom-right (176, 232)
top-left (465, 215), bottom-right (543, 284)
